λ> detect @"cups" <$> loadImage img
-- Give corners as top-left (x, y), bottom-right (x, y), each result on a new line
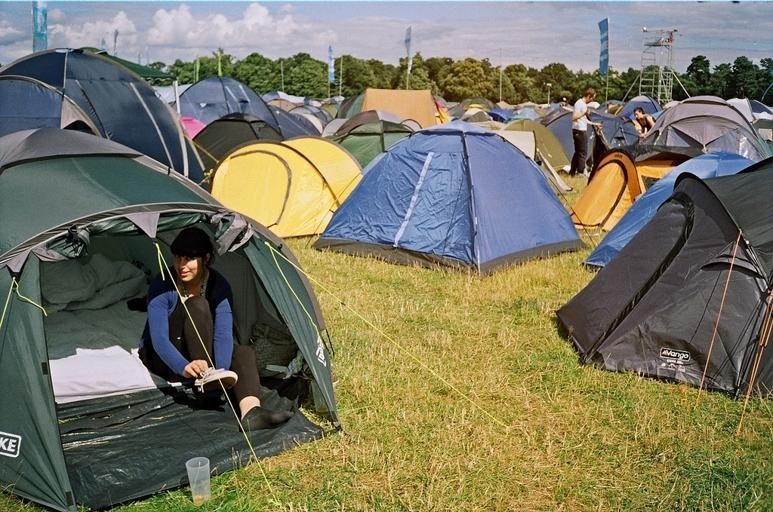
top-left (185, 456), bottom-right (212, 503)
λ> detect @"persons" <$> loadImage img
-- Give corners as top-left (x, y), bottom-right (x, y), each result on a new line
top-left (138, 227), bottom-right (294, 433)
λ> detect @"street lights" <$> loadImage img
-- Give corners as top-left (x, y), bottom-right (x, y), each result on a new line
top-left (546, 83), bottom-right (553, 105)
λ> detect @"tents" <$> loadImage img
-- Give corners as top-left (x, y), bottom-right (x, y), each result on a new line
top-left (557, 154), bottom-right (773, 399)
top-left (1, 47), bottom-right (772, 273)
top-left (0, 128), bottom-right (342, 510)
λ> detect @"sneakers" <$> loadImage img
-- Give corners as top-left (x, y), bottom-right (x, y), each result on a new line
top-left (193, 367), bottom-right (237, 393)
